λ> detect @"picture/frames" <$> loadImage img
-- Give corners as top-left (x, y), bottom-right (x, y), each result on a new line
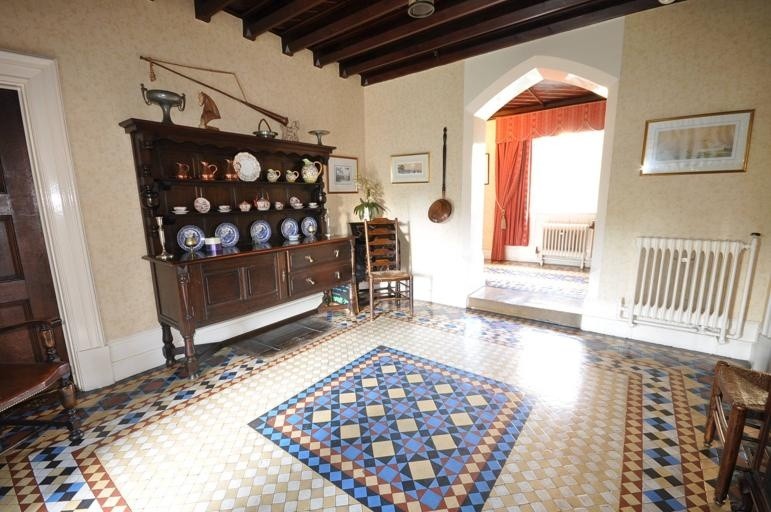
top-left (326, 155), bottom-right (359, 194)
top-left (390, 152), bottom-right (431, 183)
top-left (639, 108), bottom-right (755, 178)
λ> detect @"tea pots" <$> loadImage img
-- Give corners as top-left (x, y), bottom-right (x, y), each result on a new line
top-left (222, 159), bottom-right (241, 180)
top-left (176, 161), bottom-right (193, 181)
top-left (267, 169), bottom-right (281, 182)
top-left (301, 159), bottom-right (323, 183)
top-left (286, 170), bottom-right (299, 183)
top-left (198, 159), bottom-right (219, 180)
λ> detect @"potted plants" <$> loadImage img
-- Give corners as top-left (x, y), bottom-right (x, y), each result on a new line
top-left (353, 174), bottom-right (388, 222)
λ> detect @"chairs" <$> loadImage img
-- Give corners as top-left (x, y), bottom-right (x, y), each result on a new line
top-left (2, 314), bottom-right (84, 462)
top-left (364, 217), bottom-right (414, 321)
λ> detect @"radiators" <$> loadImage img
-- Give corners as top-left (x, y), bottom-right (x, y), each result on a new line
top-left (616, 229), bottom-right (762, 345)
top-left (533, 223), bottom-right (593, 273)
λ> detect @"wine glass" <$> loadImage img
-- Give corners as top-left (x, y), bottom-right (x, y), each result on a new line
top-left (140, 83), bottom-right (186, 125)
top-left (184, 236), bottom-right (200, 258)
top-left (308, 223), bottom-right (316, 240)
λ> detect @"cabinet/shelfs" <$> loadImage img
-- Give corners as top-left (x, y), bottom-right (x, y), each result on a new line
top-left (348, 219), bottom-right (402, 317)
top-left (116, 116), bottom-right (362, 385)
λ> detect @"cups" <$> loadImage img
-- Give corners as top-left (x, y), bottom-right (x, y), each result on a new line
top-left (169, 195), bottom-right (320, 215)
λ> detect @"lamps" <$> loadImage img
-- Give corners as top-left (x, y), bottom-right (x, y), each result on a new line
top-left (407, 0), bottom-right (437, 20)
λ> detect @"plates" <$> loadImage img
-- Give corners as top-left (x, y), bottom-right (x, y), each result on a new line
top-left (178, 238), bottom-right (319, 262)
top-left (215, 222), bottom-right (239, 247)
top-left (176, 225), bottom-right (205, 253)
top-left (301, 217), bottom-right (318, 237)
top-left (281, 218), bottom-right (299, 239)
top-left (250, 219), bottom-right (273, 243)
top-left (233, 152), bottom-right (262, 182)
